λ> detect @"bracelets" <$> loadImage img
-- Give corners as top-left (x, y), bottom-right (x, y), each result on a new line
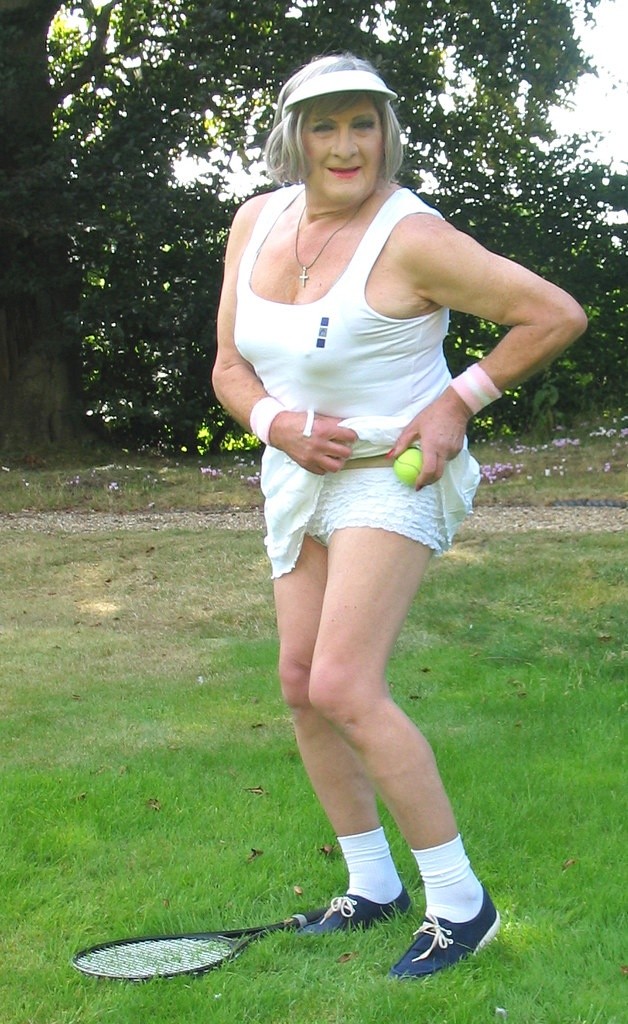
top-left (448, 363), bottom-right (502, 416)
top-left (249, 396), bottom-right (289, 447)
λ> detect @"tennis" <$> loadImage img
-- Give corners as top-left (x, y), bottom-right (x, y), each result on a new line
top-left (393, 449), bottom-right (427, 488)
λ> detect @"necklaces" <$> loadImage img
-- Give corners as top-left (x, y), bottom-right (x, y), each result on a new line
top-left (295, 200), bottom-right (361, 288)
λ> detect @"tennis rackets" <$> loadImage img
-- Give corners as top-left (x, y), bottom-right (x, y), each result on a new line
top-left (71, 908), bottom-right (327, 980)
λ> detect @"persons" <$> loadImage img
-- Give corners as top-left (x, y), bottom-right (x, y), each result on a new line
top-left (212, 55), bottom-right (587, 980)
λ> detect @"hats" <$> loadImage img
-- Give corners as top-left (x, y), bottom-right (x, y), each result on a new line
top-left (280, 70), bottom-right (398, 118)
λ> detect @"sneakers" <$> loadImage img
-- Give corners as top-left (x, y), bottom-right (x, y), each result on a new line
top-left (294, 884), bottom-right (411, 935)
top-left (387, 883), bottom-right (500, 982)
